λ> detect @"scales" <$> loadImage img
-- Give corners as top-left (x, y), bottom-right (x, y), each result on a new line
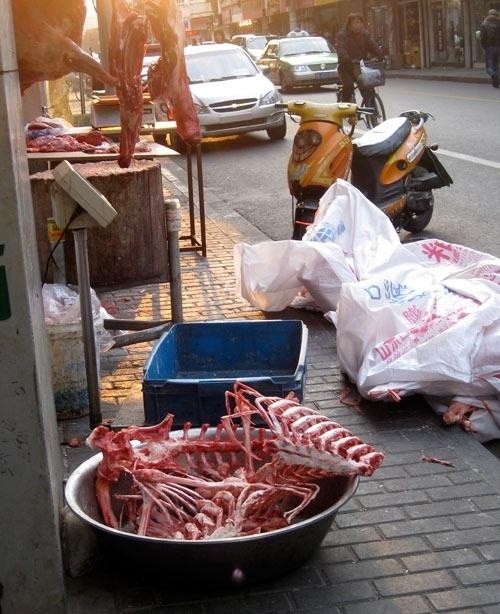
top-left (90, 93), bottom-right (155, 131)
top-left (52, 161), bottom-right (270, 431)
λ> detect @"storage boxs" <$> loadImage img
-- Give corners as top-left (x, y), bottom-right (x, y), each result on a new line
top-left (140, 317), bottom-right (310, 430)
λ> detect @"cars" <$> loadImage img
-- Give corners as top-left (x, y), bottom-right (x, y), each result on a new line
top-left (229, 31), bottom-right (278, 66)
top-left (264, 29), bottom-right (341, 90)
top-left (153, 41), bottom-right (286, 155)
top-left (141, 41), bottom-right (163, 92)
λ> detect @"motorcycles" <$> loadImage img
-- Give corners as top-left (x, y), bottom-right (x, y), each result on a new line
top-left (274, 99), bottom-right (455, 239)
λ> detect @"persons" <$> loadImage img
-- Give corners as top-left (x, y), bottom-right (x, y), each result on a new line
top-left (191, 23), bottom-right (337, 52)
top-left (480, 8), bottom-right (500, 89)
top-left (335, 12), bottom-right (384, 127)
top-left (453, 28), bottom-right (484, 64)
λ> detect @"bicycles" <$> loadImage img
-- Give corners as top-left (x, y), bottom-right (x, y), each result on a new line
top-left (336, 56), bottom-right (385, 137)
